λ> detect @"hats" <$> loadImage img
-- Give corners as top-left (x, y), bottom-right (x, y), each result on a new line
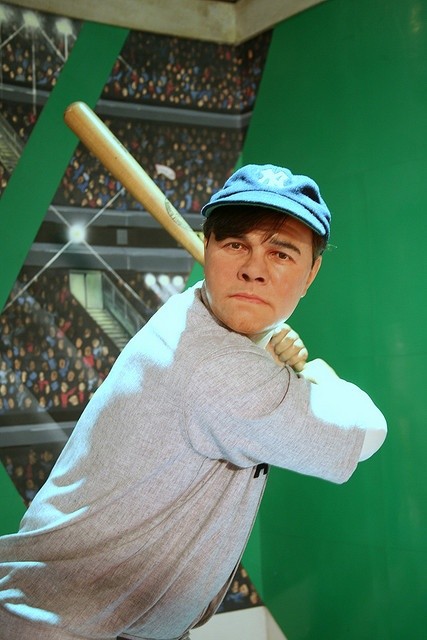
top-left (201, 165), bottom-right (331, 243)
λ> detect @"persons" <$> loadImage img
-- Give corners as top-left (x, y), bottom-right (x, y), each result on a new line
top-left (0, 164), bottom-right (388, 640)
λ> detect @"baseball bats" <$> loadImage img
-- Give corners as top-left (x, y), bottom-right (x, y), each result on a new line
top-left (63, 99), bottom-right (308, 373)
